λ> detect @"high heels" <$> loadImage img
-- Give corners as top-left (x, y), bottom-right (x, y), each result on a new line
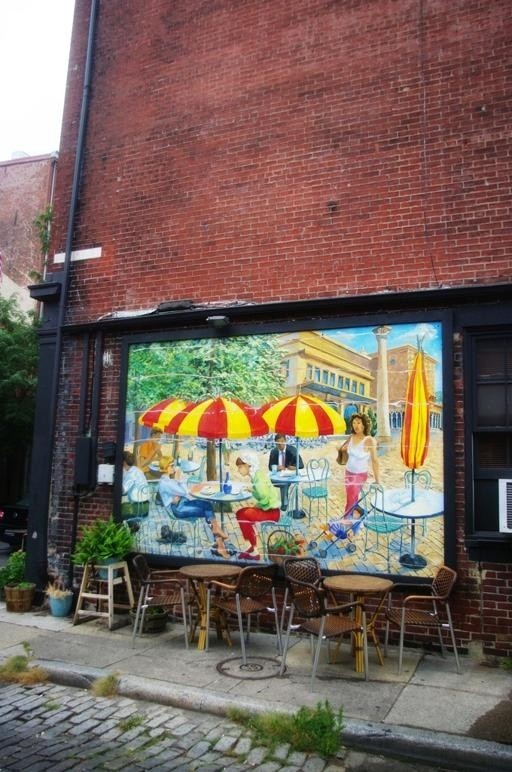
top-left (218, 549), bottom-right (231, 560)
top-left (213, 531), bottom-right (229, 542)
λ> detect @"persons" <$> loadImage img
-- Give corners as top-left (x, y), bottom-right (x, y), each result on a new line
top-left (117, 415), bottom-right (381, 559)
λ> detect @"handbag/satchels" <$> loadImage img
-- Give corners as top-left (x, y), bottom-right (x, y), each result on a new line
top-left (335, 448), bottom-right (348, 465)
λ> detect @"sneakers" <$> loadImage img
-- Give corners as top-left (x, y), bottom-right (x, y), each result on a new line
top-left (241, 553), bottom-right (260, 560)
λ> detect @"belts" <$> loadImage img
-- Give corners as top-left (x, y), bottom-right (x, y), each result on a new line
top-left (135, 500), bottom-right (149, 505)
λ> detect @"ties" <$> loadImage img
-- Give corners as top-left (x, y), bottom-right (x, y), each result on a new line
top-left (281, 450), bottom-right (285, 466)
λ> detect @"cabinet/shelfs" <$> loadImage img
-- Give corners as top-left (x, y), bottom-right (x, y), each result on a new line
top-left (72, 562), bottom-right (135, 631)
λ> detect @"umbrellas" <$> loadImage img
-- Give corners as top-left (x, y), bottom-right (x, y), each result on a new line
top-left (400, 340), bottom-right (429, 568)
top-left (254, 392), bottom-right (347, 508)
top-left (138, 397), bottom-right (269, 482)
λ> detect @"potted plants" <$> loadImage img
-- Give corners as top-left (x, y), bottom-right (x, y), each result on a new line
top-left (44, 581), bottom-right (74, 617)
top-left (128, 605), bottom-right (169, 634)
top-left (72, 513), bottom-right (136, 580)
top-left (3, 551), bottom-right (36, 612)
top-left (26, 204), bottom-right (61, 299)
